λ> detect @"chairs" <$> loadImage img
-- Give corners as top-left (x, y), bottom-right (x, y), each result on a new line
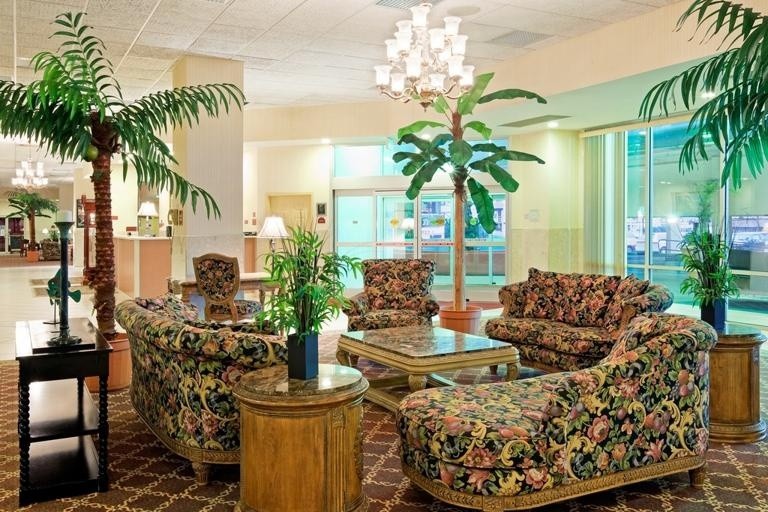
top-left (192, 253), bottom-right (263, 325)
top-left (342, 258), bottom-right (440, 366)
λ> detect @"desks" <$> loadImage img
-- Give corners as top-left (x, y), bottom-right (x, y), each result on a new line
top-left (166, 268), bottom-right (287, 337)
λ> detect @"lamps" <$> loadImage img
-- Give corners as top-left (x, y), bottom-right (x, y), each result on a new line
top-left (11, 129), bottom-right (48, 193)
top-left (257, 213), bottom-right (291, 272)
top-left (136, 201), bottom-right (158, 238)
top-left (372, 1), bottom-right (475, 112)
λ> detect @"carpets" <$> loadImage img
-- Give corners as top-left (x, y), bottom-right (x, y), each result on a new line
top-left (0, 319), bottom-right (764, 511)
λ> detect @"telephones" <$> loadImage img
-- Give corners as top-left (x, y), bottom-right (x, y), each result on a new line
top-left (168, 209), bottom-right (178, 225)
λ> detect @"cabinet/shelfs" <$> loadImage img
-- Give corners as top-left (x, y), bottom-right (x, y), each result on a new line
top-left (13, 316), bottom-right (114, 507)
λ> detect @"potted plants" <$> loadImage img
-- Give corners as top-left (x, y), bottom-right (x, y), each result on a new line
top-left (254, 218), bottom-right (366, 381)
top-left (675, 231), bottom-right (741, 329)
top-left (0, 10), bottom-right (247, 393)
top-left (392, 71), bottom-right (548, 336)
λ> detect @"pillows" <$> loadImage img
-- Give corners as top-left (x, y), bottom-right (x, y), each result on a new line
top-left (524, 266), bottom-right (651, 333)
